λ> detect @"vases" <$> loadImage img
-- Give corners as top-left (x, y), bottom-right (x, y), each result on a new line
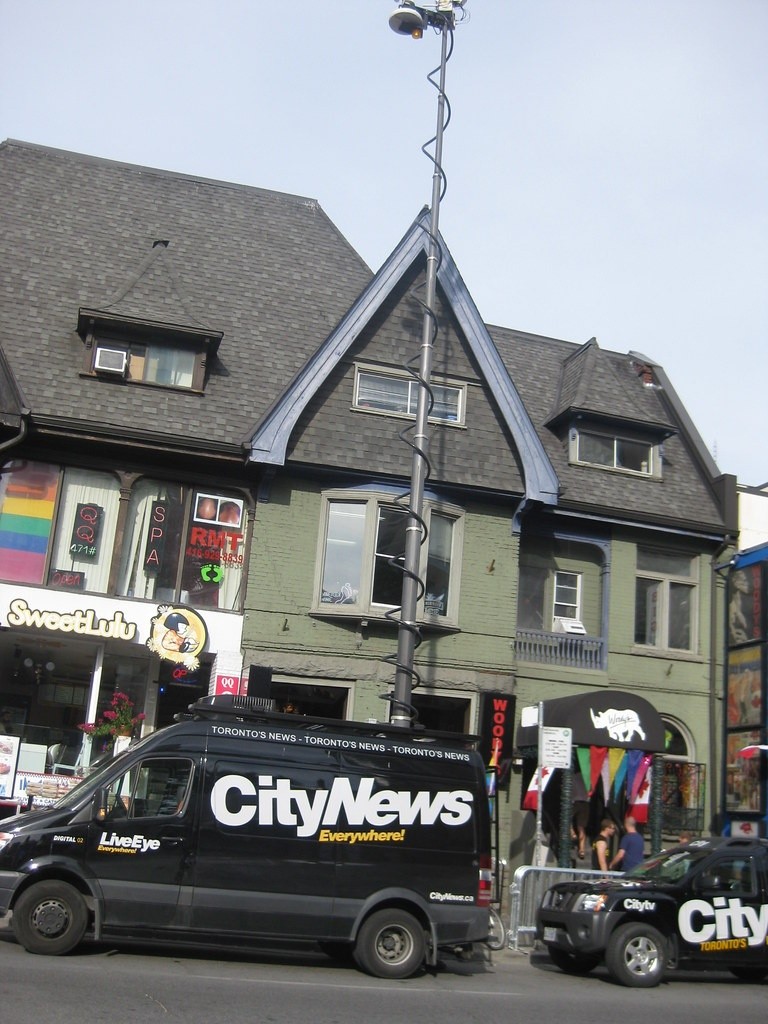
top-left (117, 724), bottom-right (134, 737)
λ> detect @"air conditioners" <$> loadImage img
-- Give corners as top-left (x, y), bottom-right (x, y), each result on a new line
top-left (94, 347), bottom-right (129, 378)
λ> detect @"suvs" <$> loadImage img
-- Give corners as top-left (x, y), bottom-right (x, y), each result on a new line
top-left (535, 835), bottom-right (768, 986)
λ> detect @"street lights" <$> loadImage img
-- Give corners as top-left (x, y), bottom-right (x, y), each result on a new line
top-left (388, 1), bottom-right (457, 724)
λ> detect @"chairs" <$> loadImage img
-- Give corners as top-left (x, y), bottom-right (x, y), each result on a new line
top-left (175, 785), bottom-right (186, 806)
top-left (45, 743), bottom-right (85, 777)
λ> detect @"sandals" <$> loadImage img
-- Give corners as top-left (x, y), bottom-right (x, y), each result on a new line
top-left (579, 851), bottom-right (584, 858)
top-left (570, 833), bottom-right (577, 841)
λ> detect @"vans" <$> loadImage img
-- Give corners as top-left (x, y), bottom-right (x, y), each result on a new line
top-left (1, 704), bottom-right (502, 979)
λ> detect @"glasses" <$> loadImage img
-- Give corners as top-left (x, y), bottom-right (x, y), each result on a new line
top-left (608, 826), bottom-right (615, 830)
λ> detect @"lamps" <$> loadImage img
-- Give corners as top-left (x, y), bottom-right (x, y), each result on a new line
top-left (5, 637), bottom-right (54, 687)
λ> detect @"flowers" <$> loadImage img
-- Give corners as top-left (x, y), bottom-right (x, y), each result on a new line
top-left (78, 684), bottom-right (147, 753)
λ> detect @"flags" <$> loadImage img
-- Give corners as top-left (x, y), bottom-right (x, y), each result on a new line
top-left (626, 767), bottom-right (652, 823)
top-left (523, 765), bottom-right (554, 810)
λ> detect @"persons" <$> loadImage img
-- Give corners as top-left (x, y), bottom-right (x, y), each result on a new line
top-left (609, 817), bottom-right (645, 872)
top-left (571, 772), bottom-right (590, 857)
top-left (591, 820), bottom-right (617, 871)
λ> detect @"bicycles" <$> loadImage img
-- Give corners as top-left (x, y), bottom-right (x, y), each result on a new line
top-left (486, 906), bottom-right (506, 951)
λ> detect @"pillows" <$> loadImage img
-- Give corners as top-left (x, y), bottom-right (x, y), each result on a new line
top-left (47, 744), bottom-right (62, 764)
